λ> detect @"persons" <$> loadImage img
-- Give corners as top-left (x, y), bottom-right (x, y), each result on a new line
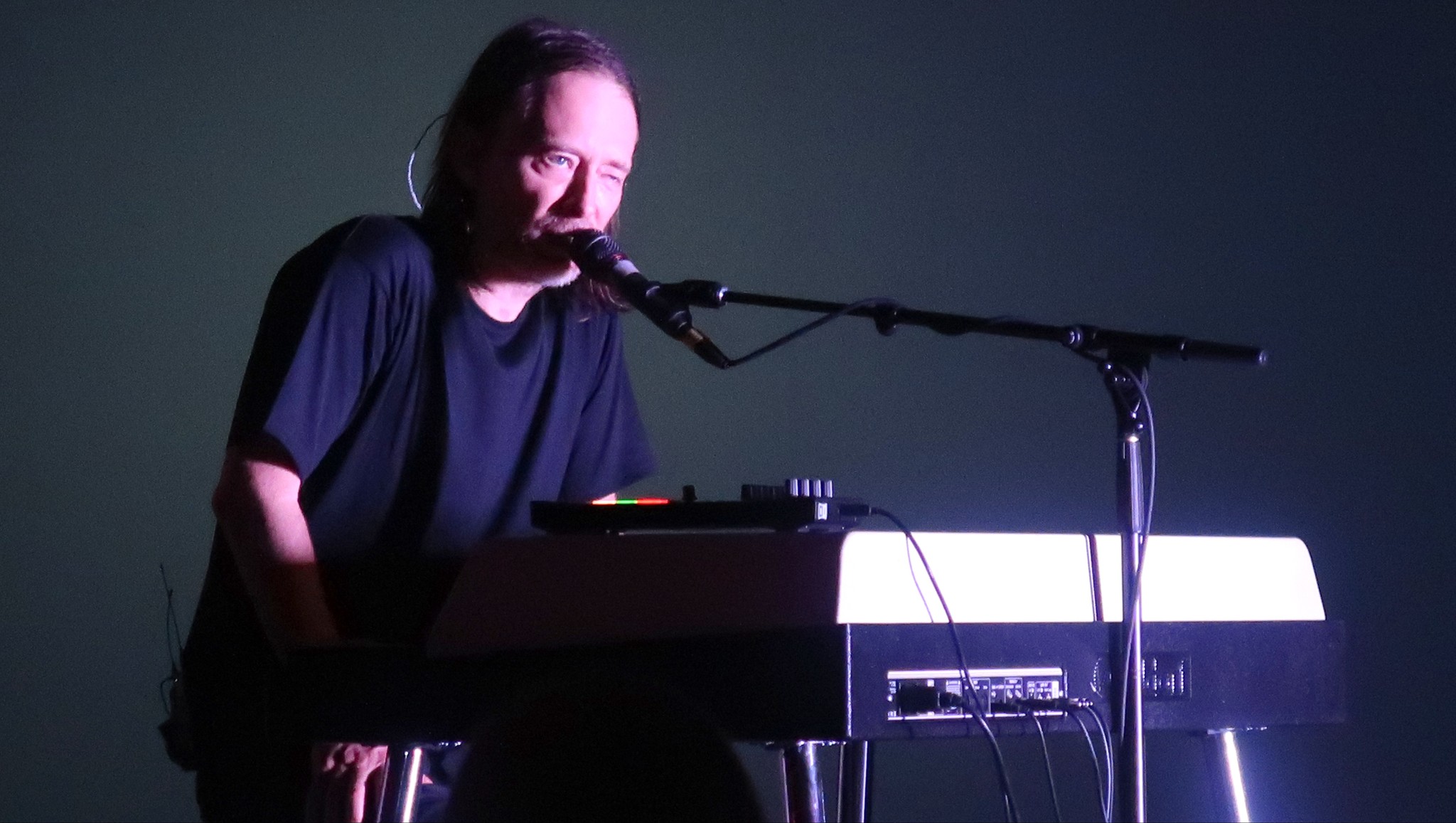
top-left (150, 19), bottom-right (665, 822)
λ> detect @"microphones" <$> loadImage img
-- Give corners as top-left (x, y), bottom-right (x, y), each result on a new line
top-left (569, 228), bottom-right (731, 373)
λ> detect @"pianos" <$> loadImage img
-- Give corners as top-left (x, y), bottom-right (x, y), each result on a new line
top-left (404, 490), bottom-right (1351, 755)
